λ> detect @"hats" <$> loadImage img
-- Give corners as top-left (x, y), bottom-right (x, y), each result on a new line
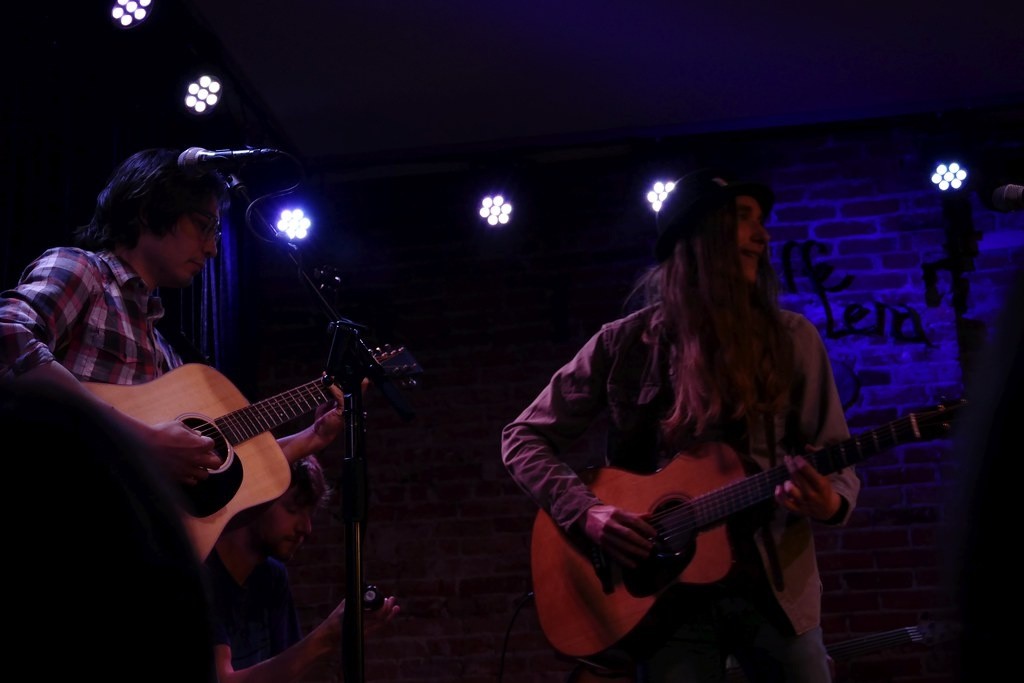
top-left (653, 163), bottom-right (774, 260)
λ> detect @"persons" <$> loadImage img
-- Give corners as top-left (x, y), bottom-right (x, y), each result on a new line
top-left (0, 147), bottom-right (368, 681)
top-left (499, 173), bottom-right (863, 683)
top-left (182, 449), bottom-right (401, 681)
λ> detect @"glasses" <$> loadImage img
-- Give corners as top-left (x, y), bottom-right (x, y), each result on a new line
top-left (194, 206), bottom-right (222, 243)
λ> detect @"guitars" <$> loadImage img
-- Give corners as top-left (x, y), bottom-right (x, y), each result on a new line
top-left (78, 341), bottom-right (425, 569)
top-left (532, 394), bottom-right (973, 657)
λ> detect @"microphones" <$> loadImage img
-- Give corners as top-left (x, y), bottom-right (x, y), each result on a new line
top-left (178, 147), bottom-right (283, 177)
